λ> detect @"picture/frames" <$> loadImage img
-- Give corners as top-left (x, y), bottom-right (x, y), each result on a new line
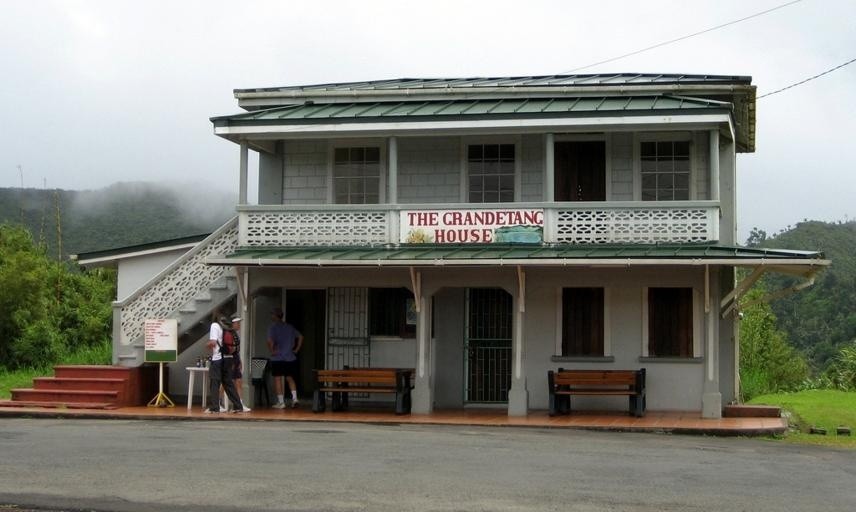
top-left (398, 292), bottom-right (432, 338)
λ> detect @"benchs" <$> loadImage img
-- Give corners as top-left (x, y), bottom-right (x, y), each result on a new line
top-left (549, 366), bottom-right (648, 418)
top-left (312, 365), bottom-right (417, 417)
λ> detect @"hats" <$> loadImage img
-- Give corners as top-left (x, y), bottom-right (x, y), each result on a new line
top-left (231, 312), bottom-right (243, 322)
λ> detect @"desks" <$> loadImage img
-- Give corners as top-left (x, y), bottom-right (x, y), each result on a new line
top-left (186, 365), bottom-right (229, 412)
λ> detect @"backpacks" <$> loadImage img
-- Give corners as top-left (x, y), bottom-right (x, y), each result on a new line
top-left (217, 320), bottom-right (240, 355)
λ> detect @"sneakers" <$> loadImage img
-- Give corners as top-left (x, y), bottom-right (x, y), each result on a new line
top-left (291, 399), bottom-right (299, 409)
top-left (272, 403), bottom-right (285, 409)
top-left (204, 407), bottom-right (226, 414)
top-left (231, 406), bottom-right (250, 413)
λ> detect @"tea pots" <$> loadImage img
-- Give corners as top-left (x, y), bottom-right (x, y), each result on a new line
top-left (205, 356), bottom-right (212, 367)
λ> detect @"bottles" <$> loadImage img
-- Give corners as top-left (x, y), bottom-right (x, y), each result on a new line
top-left (196, 356), bottom-right (200, 367)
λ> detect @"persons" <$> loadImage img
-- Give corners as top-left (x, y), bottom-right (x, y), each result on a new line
top-left (204, 309), bottom-right (251, 414)
top-left (266, 308), bottom-right (305, 409)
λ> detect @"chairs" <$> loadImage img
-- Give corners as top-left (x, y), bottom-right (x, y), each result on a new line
top-left (249, 357), bottom-right (271, 408)
